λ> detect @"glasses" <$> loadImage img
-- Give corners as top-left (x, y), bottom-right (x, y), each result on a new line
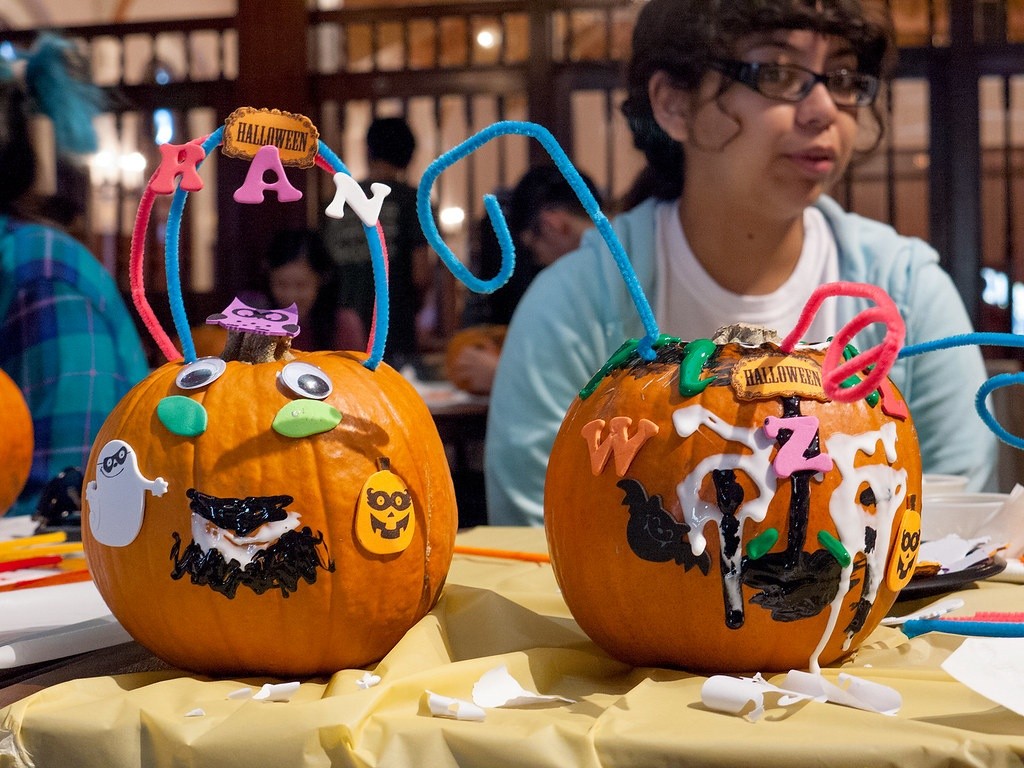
top-left (706, 56), bottom-right (882, 107)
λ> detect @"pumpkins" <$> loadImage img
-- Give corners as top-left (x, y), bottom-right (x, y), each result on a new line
top-left (446, 324), bottom-right (511, 394)
top-left (543, 322), bottom-right (923, 675)
top-left (80, 326), bottom-right (458, 679)
top-left (0, 368), bottom-right (35, 516)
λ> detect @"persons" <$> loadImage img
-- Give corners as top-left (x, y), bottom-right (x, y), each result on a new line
top-left (0, 79), bottom-right (151, 523)
top-left (240, 114), bottom-right (603, 392)
top-left (484, 1), bottom-right (997, 528)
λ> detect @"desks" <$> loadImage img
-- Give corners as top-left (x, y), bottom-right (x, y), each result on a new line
top-left (0, 513), bottom-right (1024, 768)
